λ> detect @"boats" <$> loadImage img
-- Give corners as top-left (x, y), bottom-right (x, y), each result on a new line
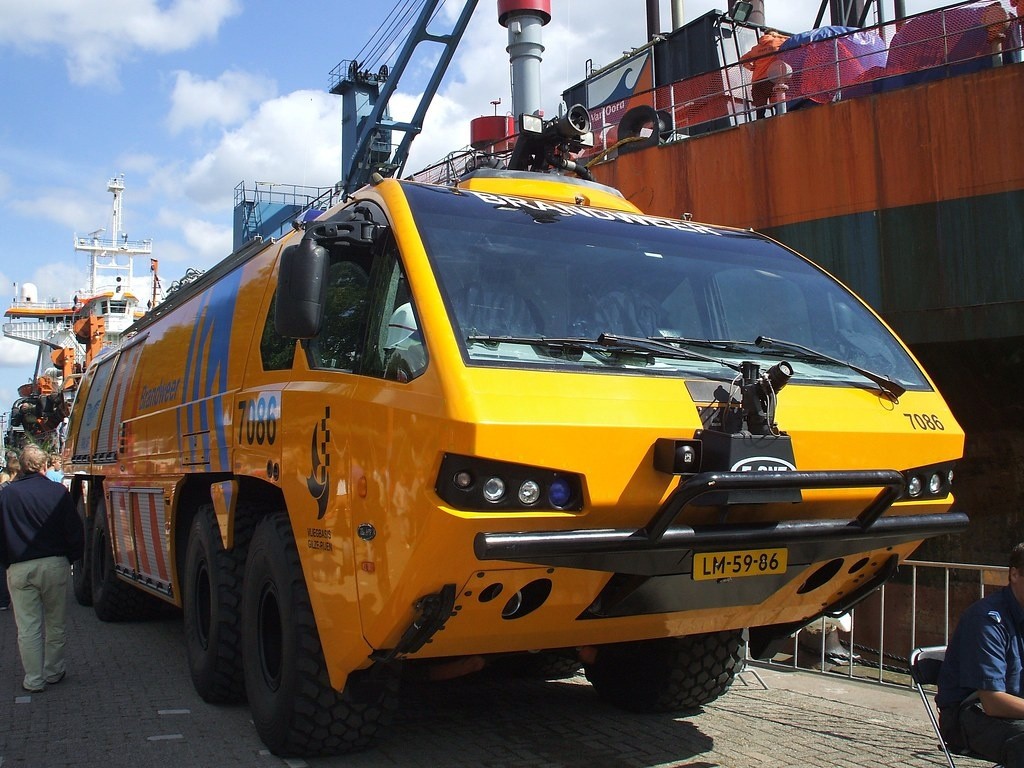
top-left (2, 171), bottom-right (174, 454)
top-left (232, 0), bottom-right (1023, 584)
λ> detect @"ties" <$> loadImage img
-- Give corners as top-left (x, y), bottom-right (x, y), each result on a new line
top-left (523, 296), bottom-right (546, 334)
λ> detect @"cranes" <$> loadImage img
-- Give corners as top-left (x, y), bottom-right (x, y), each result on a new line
top-left (231, 0), bottom-right (480, 254)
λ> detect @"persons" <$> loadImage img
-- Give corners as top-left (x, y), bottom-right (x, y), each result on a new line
top-left (0, 443), bottom-right (85, 693)
top-left (739, 28), bottom-right (791, 120)
top-left (934, 542), bottom-right (1024, 768)
top-left (0, 450), bottom-right (64, 610)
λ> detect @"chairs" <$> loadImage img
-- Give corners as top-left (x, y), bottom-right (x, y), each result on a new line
top-left (910, 645), bottom-right (1004, 768)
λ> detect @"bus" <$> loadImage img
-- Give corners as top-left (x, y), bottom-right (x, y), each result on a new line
top-left (54, 0), bottom-right (969, 764)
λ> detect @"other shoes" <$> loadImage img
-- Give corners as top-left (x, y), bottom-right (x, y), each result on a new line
top-left (22, 681), bottom-right (44, 692)
top-left (0, 606), bottom-right (8, 610)
top-left (47, 663), bottom-right (66, 684)
